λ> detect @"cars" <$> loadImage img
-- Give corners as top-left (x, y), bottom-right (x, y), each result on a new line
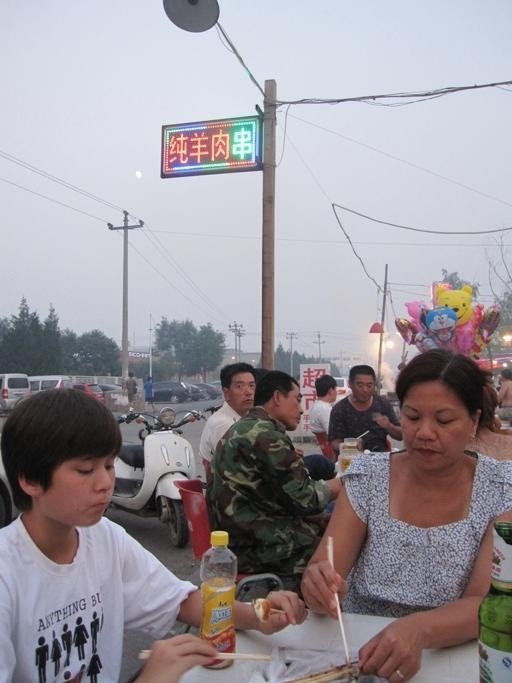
top-left (475, 352), bottom-right (512, 375)
top-left (331, 375), bottom-right (348, 406)
top-left (1, 372), bottom-right (226, 410)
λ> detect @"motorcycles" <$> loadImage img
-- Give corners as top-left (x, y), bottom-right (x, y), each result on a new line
top-left (103, 401), bottom-right (224, 547)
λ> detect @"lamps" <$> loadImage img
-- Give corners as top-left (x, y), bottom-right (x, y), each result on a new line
top-left (367, 281), bottom-right (387, 337)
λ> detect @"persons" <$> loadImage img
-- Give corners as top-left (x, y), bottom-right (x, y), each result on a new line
top-left (198, 361), bottom-right (305, 473)
top-left (205, 369), bottom-right (344, 597)
top-left (497, 369), bottom-right (511, 407)
top-left (327, 363), bottom-right (403, 452)
top-left (124, 371), bottom-right (139, 413)
top-left (309, 374), bottom-right (337, 434)
top-left (142, 375), bottom-right (156, 412)
top-left (298, 348), bottom-right (511, 682)
top-left (252, 366), bottom-right (269, 383)
top-left (0, 387), bottom-right (309, 682)
top-left (464, 384), bottom-right (512, 460)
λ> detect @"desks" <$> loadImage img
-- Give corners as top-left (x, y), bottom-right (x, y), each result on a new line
top-left (162, 599), bottom-right (487, 683)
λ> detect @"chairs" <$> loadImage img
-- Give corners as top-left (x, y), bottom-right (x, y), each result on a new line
top-left (333, 439), bottom-right (393, 464)
top-left (310, 427), bottom-right (333, 461)
top-left (198, 451), bottom-right (213, 484)
top-left (172, 473), bottom-right (287, 634)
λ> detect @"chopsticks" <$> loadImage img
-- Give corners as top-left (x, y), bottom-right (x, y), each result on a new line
top-left (139, 650), bottom-right (273, 662)
top-left (357, 430), bottom-right (369, 439)
top-left (324, 535), bottom-right (352, 667)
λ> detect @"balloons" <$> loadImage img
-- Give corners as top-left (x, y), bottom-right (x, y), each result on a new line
top-left (394, 280), bottom-right (501, 357)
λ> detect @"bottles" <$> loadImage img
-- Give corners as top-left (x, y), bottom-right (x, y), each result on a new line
top-left (199, 529), bottom-right (239, 667)
top-left (478, 519), bottom-right (511, 682)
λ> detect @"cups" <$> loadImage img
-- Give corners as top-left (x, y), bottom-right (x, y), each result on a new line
top-left (339, 437), bottom-right (363, 454)
top-left (342, 449), bottom-right (361, 472)
top-left (371, 412), bottom-right (381, 425)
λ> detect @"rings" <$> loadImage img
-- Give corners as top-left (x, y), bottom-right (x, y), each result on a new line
top-left (395, 669), bottom-right (405, 680)
